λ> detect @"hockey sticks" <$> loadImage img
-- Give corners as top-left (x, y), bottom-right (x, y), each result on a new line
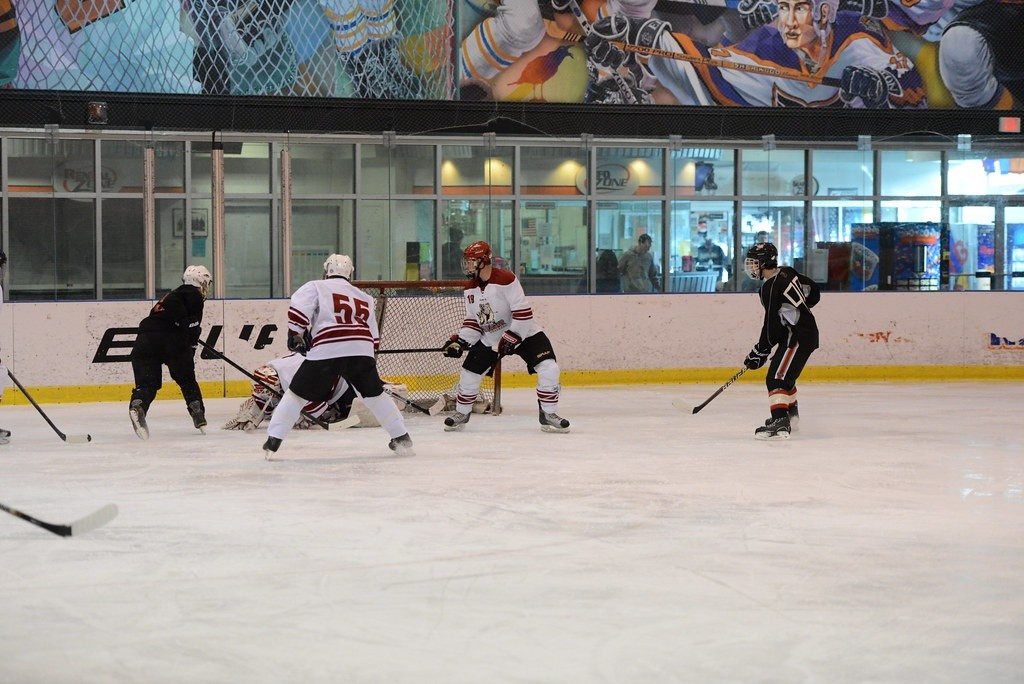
top-left (0, 500), bottom-right (119, 540)
top-left (670, 364), bottom-right (750, 414)
top-left (382, 386), bottom-right (447, 417)
top-left (198, 339), bottom-right (362, 431)
top-left (374, 346), bottom-right (472, 355)
top-left (7, 368), bottom-right (92, 444)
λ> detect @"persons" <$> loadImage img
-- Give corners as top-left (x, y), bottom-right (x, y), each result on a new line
top-left (0, 250), bottom-right (11, 444)
top-left (698, 232), bottom-right (731, 281)
top-left (576, 249), bottom-right (624, 293)
top-left (222, 365), bottom-right (491, 432)
top-left (618, 234), bottom-right (662, 293)
top-left (742, 230), bottom-right (769, 292)
top-left (130, 266), bottom-right (213, 441)
top-left (441, 241), bottom-right (570, 434)
top-left (745, 243), bottom-right (819, 440)
top-left (441, 229), bottom-right (465, 279)
top-left (262, 254), bottom-right (413, 461)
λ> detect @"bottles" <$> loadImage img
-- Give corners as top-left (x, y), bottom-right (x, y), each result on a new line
top-left (708, 259), bottom-right (714, 273)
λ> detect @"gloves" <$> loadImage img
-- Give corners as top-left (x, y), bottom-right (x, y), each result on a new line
top-left (179, 317), bottom-right (201, 347)
top-left (442, 334), bottom-right (467, 358)
top-left (286, 329), bottom-right (312, 357)
top-left (743, 341), bottom-right (773, 370)
top-left (496, 329), bottom-right (521, 356)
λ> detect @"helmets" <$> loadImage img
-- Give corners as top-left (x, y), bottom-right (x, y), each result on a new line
top-left (322, 254), bottom-right (352, 279)
top-left (460, 240), bottom-right (490, 277)
top-left (743, 241), bottom-right (778, 280)
top-left (181, 264), bottom-right (212, 290)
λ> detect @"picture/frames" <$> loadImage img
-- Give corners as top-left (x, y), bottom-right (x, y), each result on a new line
top-left (173, 208), bottom-right (208, 237)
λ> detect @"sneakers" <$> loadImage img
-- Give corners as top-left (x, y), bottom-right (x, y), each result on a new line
top-left (444, 409), bottom-right (469, 431)
top-left (537, 398), bottom-right (570, 433)
top-left (755, 412), bottom-right (791, 439)
top-left (1, 429), bottom-right (10, 445)
top-left (387, 434), bottom-right (415, 457)
top-left (765, 403), bottom-right (799, 429)
top-left (188, 402), bottom-right (207, 433)
top-left (130, 407), bottom-right (149, 441)
top-left (262, 436), bottom-right (283, 461)
